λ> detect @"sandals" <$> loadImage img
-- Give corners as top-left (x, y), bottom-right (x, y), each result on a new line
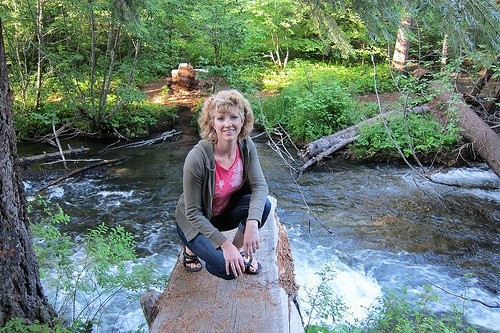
top-left (182, 244), bottom-right (202, 272)
top-left (236, 250), bottom-right (261, 274)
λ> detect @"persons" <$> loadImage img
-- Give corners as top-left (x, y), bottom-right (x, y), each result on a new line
top-left (174, 89), bottom-right (272, 281)
